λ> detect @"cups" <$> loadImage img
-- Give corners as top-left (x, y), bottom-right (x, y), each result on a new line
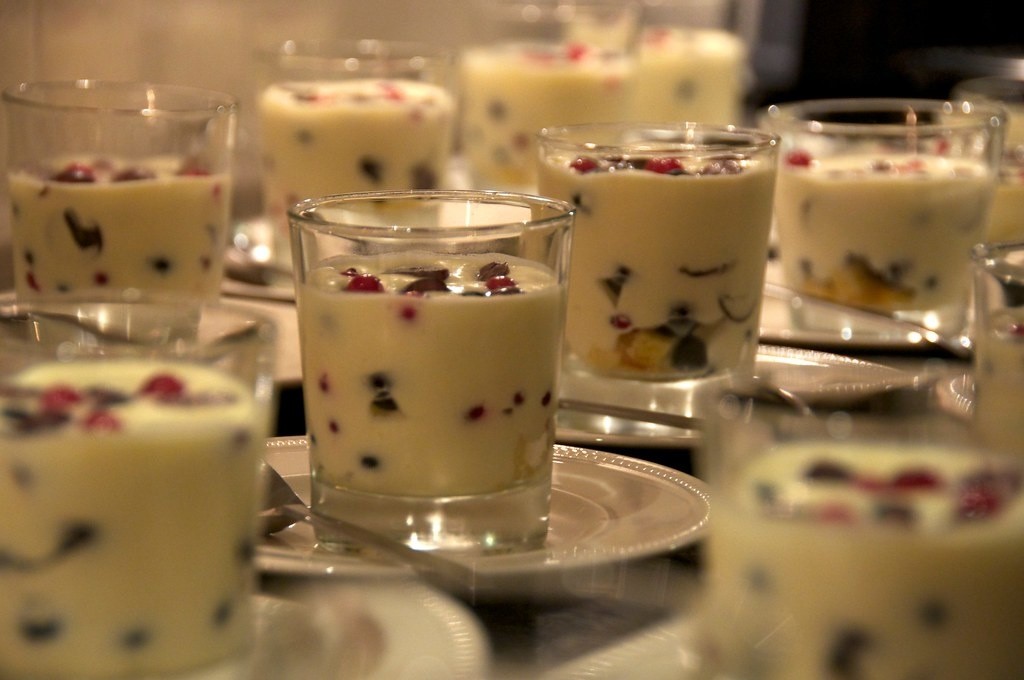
top-left (252, 37), bottom-right (463, 284)
top-left (284, 190), bottom-right (579, 555)
top-left (463, 32), bottom-right (633, 202)
top-left (971, 241), bottom-right (1024, 444)
top-left (953, 73), bottom-right (1024, 249)
top-left (755, 98), bottom-right (1002, 336)
top-left (626, 26), bottom-right (746, 143)
top-left (2, 80), bottom-right (244, 343)
top-left (694, 376), bottom-right (1024, 680)
top-left (535, 123), bottom-right (781, 408)
top-left (0, 289), bottom-right (278, 680)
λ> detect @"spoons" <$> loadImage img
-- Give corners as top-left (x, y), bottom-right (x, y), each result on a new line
top-left (242, 457), bottom-right (480, 581)
top-left (229, 243), bottom-right (298, 294)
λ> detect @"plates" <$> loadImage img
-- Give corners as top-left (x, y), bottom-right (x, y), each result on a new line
top-left (227, 542), bottom-right (493, 680)
top-left (550, 342), bottom-right (928, 459)
top-left (251, 432), bottom-right (720, 589)
top-left (217, 201), bottom-right (312, 299)
top-left (178, 291), bottom-right (301, 391)
top-left (538, 612), bottom-right (694, 680)
top-left (738, 252), bottom-right (1011, 356)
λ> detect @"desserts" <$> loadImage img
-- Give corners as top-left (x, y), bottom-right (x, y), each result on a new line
top-left (0, 0), bottom-right (1024, 680)
